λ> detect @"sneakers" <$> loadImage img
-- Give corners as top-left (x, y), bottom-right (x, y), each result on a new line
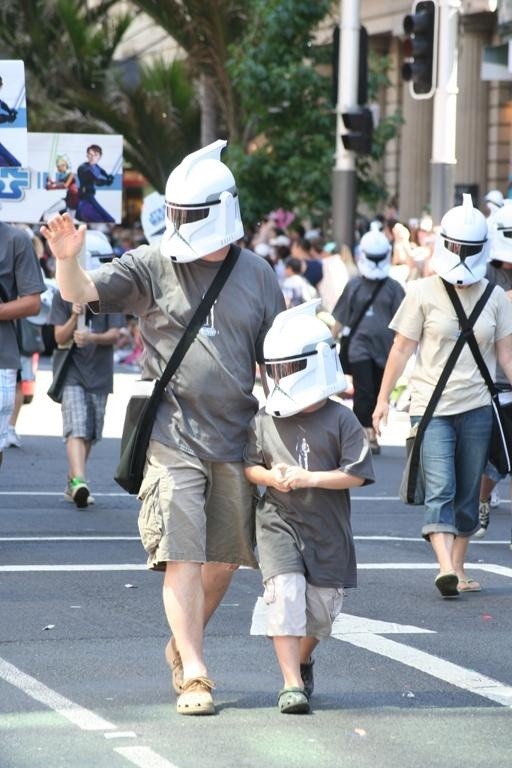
top-left (369, 439), bottom-right (379, 453)
top-left (277, 686), bottom-right (311, 715)
top-left (489, 484), bottom-right (500, 508)
top-left (165, 636), bottom-right (185, 695)
top-left (64, 471), bottom-right (96, 508)
top-left (299, 654), bottom-right (316, 695)
top-left (176, 676), bottom-right (217, 715)
top-left (473, 495), bottom-right (491, 538)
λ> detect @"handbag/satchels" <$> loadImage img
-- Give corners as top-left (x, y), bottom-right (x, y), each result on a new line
top-left (47, 337), bottom-right (76, 403)
top-left (113, 379), bottom-right (161, 495)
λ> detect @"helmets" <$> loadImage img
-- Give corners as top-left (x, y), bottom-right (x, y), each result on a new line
top-left (357, 221), bottom-right (392, 280)
top-left (142, 190), bottom-right (167, 246)
top-left (429, 190), bottom-right (511, 287)
top-left (55, 156), bottom-right (71, 181)
top-left (75, 229), bottom-right (115, 272)
top-left (338, 334), bottom-right (350, 375)
top-left (263, 297), bottom-right (347, 418)
top-left (399, 435), bottom-right (427, 506)
top-left (158, 139), bottom-right (245, 264)
top-left (487, 397), bottom-right (512, 479)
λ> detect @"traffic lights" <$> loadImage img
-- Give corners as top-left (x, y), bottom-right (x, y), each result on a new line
top-left (341, 106), bottom-right (374, 150)
top-left (401, 1), bottom-right (436, 94)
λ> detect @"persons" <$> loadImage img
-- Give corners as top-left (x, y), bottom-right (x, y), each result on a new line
top-left (240, 302), bottom-right (378, 715)
top-left (40, 140), bottom-right (287, 713)
top-left (0, 189), bottom-right (512, 600)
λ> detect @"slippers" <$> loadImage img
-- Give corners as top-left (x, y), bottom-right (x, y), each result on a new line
top-left (434, 570), bottom-right (481, 596)
